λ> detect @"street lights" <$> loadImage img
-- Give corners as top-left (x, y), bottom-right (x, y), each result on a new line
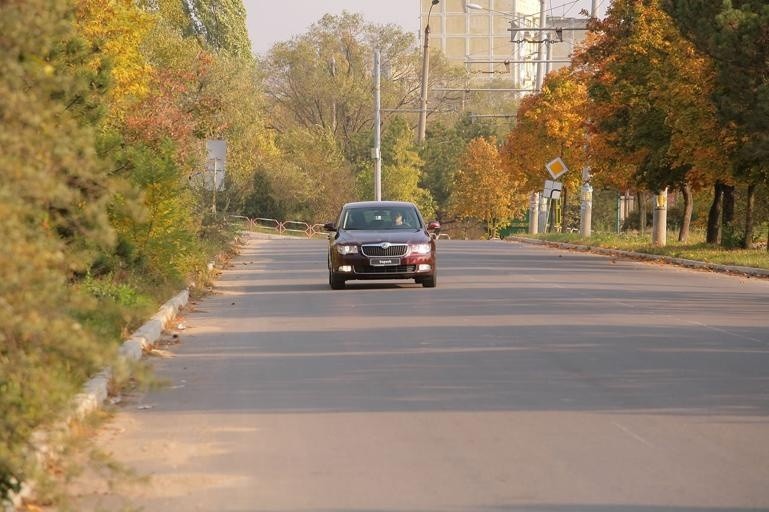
top-left (417, 0), bottom-right (441, 141)
top-left (464, 3), bottom-right (554, 97)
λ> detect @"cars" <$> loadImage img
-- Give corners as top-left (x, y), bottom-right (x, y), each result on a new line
top-left (323, 201), bottom-right (441, 290)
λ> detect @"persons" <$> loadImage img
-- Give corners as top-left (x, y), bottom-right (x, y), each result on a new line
top-left (390, 209), bottom-right (410, 229)
